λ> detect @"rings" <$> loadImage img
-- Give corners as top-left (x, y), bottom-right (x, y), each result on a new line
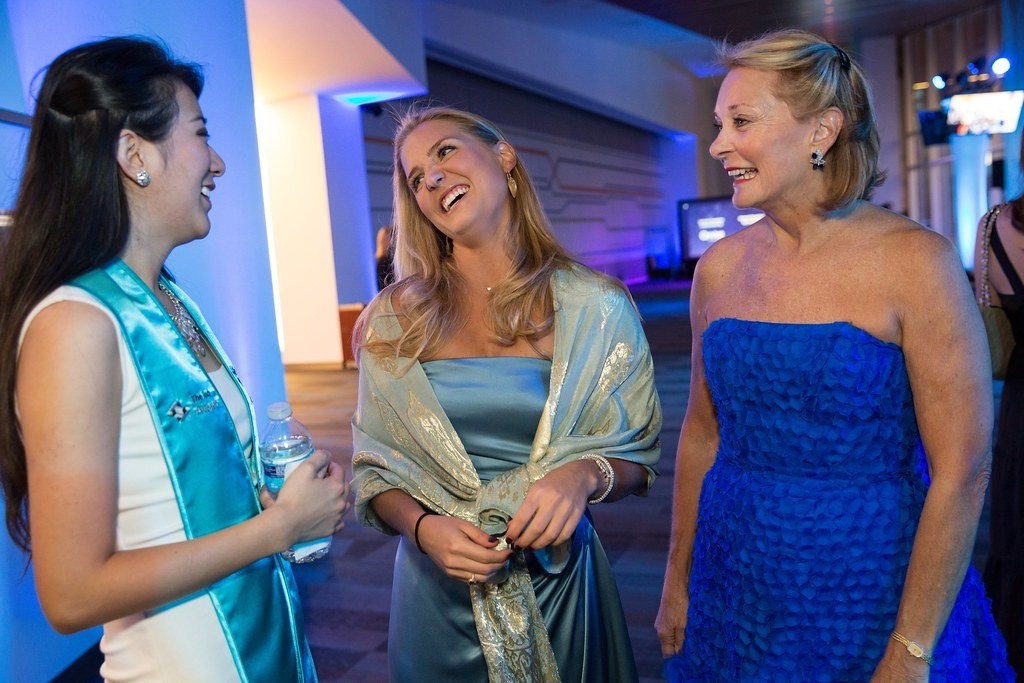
top-left (468, 573), bottom-right (477, 585)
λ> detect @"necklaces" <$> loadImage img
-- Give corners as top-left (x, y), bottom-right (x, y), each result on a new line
top-left (158, 284), bottom-right (207, 361)
top-left (486, 286), bottom-right (492, 291)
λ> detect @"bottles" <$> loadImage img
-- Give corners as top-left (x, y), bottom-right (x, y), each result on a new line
top-left (257, 401), bottom-right (334, 564)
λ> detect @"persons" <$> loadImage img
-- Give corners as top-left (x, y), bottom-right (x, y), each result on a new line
top-left (653, 29), bottom-right (1019, 683)
top-left (375, 227), bottom-right (396, 293)
top-left (350, 102), bottom-right (664, 683)
top-left (974, 124), bottom-right (1024, 683)
top-left (0, 33), bottom-right (348, 683)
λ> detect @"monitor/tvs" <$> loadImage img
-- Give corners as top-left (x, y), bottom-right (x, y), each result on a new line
top-left (677, 195), bottom-right (766, 262)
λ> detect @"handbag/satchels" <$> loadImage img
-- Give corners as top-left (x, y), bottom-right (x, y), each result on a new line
top-left (976, 202), bottom-right (1015, 381)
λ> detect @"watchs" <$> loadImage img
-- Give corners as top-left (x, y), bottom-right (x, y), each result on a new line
top-left (890, 630), bottom-right (933, 666)
top-left (591, 457), bottom-right (610, 497)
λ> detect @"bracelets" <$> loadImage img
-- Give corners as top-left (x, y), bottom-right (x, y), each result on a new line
top-left (577, 453), bottom-right (614, 504)
top-left (415, 512), bottom-right (440, 555)
